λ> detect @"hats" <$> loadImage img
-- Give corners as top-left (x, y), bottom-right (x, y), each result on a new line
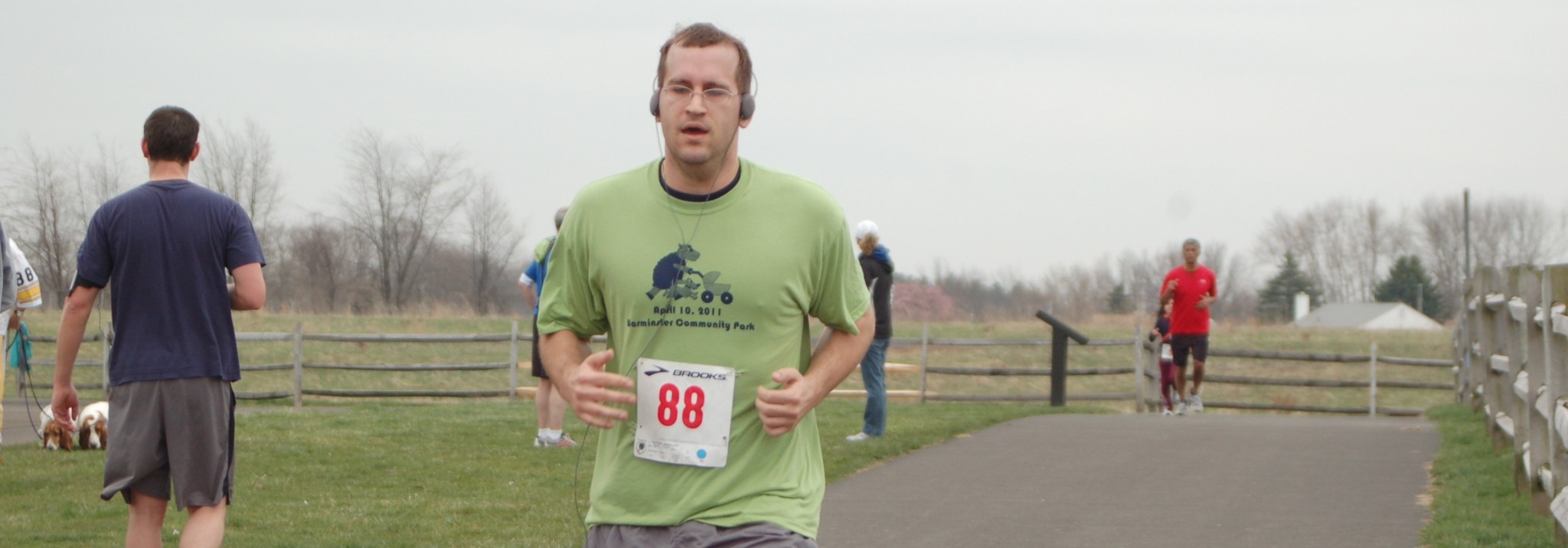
top-left (854, 220), bottom-right (878, 240)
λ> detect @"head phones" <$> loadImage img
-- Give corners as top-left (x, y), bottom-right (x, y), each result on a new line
top-left (650, 66), bottom-right (760, 120)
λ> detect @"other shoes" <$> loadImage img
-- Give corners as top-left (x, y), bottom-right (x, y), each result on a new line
top-left (846, 432), bottom-right (870, 442)
top-left (1190, 386), bottom-right (1203, 411)
top-left (1177, 402), bottom-right (1189, 415)
top-left (1173, 391), bottom-right (1181, 403)
top-left (1163, 410), bottom-right (1172, 415)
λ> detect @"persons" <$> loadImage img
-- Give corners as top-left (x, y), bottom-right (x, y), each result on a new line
top-left (537, 22), bottom-right (876, 548)
top-left (1159, 238), bottom-right (1217, 412)
top-left (52, 106), bottom-right (266, 548)
top-left (1149, 297), bottom-right (1180, 415)
top-left (845, 231), bottom-right (894, 439)
top-left (519, 206), bottom-right (577, 445)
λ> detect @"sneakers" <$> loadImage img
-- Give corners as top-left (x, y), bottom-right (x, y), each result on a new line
top-left (535, 432), bottom-right (577, 448)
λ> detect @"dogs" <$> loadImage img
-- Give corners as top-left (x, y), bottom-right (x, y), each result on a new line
top-left (38, 404), bottom-right (78, 451)
top-left (78, 401), bottom-right (109, 451)
